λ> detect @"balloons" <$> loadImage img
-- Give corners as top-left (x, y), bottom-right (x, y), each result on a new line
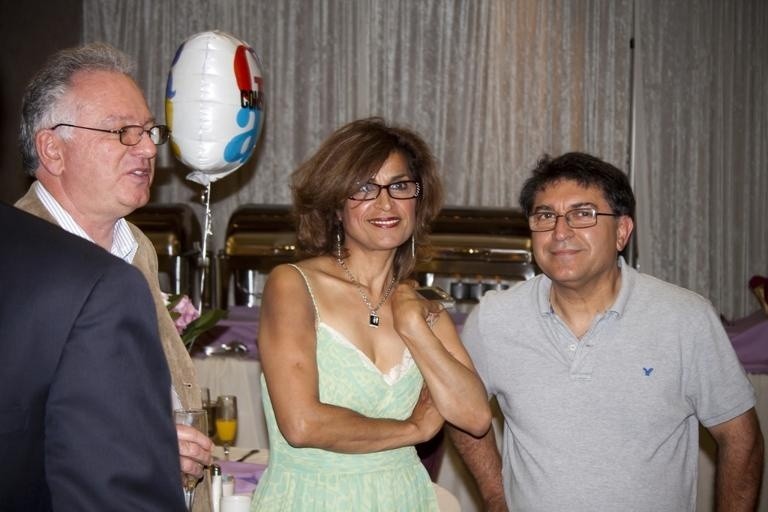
top-left (163, 29), bottom-right (265, 190)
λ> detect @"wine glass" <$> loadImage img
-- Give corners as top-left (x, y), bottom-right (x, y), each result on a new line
top-left (173, 410), bottom-right (208, 512)
top-left (214, 395), bottom-right (241, 461)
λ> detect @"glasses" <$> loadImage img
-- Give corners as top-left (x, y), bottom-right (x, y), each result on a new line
top-left (47, 122), bottom-right (172, 147)
top-left (528, 209), bottom-right (619, 233)
top-left (343, 175), bottom-right (422, 201)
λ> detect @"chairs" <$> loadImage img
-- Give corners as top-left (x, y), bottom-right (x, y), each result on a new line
top-left (122, 203), bottom-right (547, 304)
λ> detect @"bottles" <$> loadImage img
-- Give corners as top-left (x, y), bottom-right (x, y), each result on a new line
top-left (211, 464), bottom-right (222, 512)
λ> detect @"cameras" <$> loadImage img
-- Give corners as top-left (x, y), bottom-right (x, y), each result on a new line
top-left (413, 285), bottom-right (456, 310)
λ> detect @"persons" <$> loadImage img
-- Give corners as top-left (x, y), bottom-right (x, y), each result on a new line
top-left (246, 114), bottom-right (493, 512)
top-left (10, 39), bottom-right (215, 512)
top-left (444, 151), bottom-right (764, 512)
top-left (0, 199), bottom-right (191, 512)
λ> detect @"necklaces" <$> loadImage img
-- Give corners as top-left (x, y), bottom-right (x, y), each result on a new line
top-left (335, 253), bottom-right (398, 329)
